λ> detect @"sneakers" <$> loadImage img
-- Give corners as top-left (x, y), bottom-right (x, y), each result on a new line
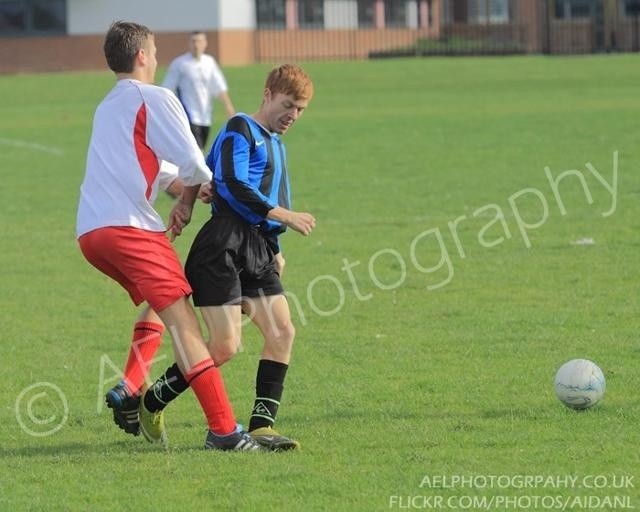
top-left (105, 381), bottom-right (142, 436)
top-left (140, 383), bottom-right (164, 443)
top-left (204, 424), bottom-right (264, 450)
top-left (250, 424), bottom-right (298, 450)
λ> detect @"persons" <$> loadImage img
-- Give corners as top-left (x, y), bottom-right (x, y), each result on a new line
top-left (157, 30), bottom-right (237, 157)
top-left (75, 21), bottom-right (266, 452)
top-left (136, 64), bottom-right (315, 453)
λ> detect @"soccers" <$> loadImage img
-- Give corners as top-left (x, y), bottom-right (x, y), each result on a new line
top-left (555, 359), bottom-right (605, 409)
